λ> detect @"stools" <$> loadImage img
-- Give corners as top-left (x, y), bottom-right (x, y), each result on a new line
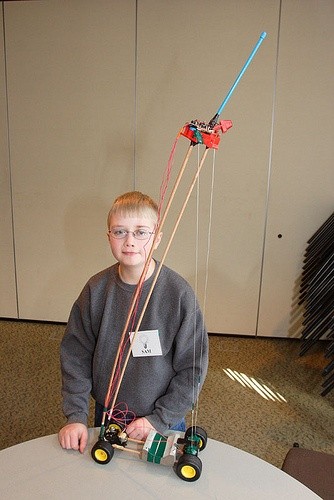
top-left (281, 443), bottom-right (334, 500)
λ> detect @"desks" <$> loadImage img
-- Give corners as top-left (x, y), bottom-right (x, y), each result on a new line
top-left (0, 426), bottom-right (324, 500)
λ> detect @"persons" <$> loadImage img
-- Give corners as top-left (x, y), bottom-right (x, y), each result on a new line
top-left (59, 189), bottom-right (211, 454)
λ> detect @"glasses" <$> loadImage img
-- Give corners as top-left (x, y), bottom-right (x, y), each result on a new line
top-left (108, 228), bottom-right (153, 240)
